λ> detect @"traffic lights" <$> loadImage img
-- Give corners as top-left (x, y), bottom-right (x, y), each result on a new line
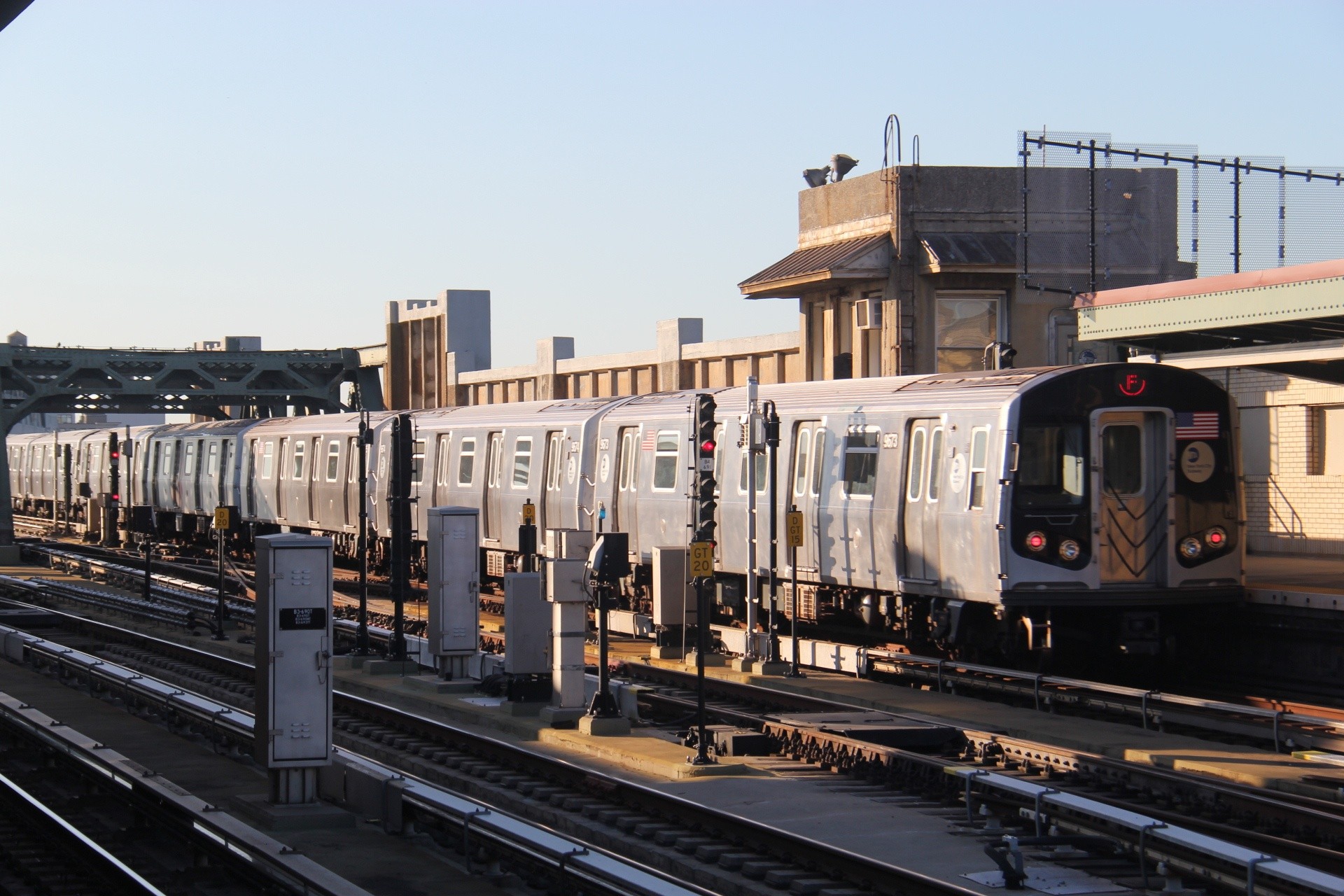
top-left (696, 393), bottom-right (717, 556)
top-left (995, 343), bottom-right (1018, 370)
top-left (110, 432), bottom-right (119, 508)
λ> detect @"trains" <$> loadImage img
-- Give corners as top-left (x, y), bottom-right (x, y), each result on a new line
top-left (6, 358), bottom-right (1248, 665)
